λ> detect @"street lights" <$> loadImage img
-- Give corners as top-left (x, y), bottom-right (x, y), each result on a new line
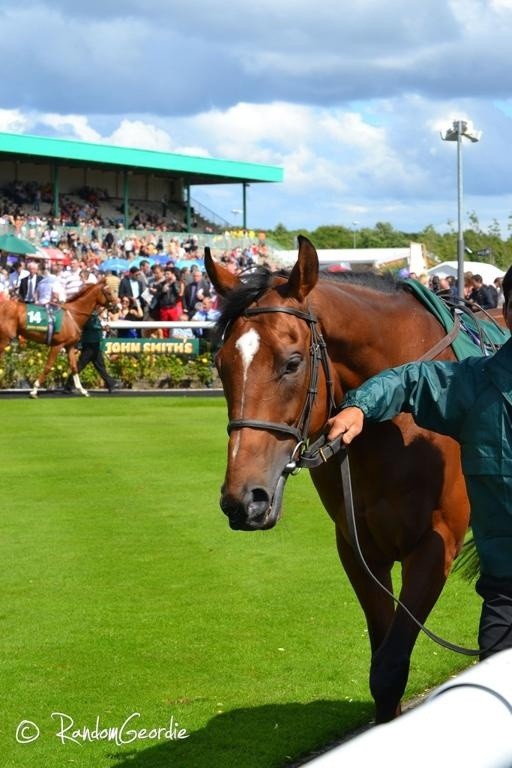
top-left (436, 120), bottom-right (482, 308)
top-left (231, 209), bottom-right (243, 228)
top-left (352, 221), bottom-right (359, 248)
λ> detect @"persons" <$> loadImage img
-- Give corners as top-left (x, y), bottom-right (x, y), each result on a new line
top-left (327, 262), bottom-right (512, 657)
top-left (61, 309), bottom-right (121, 395)
top-left (0, 173), bottom-right (512, 346)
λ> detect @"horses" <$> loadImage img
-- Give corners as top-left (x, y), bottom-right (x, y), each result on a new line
top-left (203, 234), bottom-right (512, 726)
top-left (0, 277), bottom-right (121, 399)
